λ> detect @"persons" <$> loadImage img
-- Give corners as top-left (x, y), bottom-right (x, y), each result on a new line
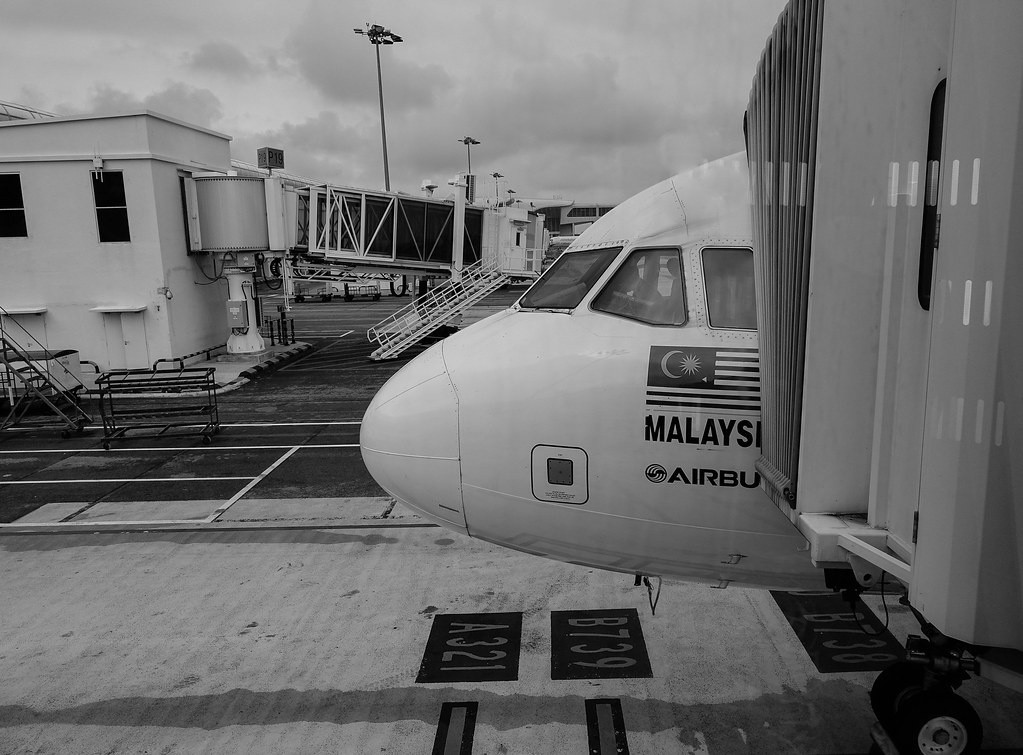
top-left (292, 256), bottom-right (298, 267)
top-left (344, 283), bottom-right (349, 295)
top-left (613, 265), bottom-right (664, 309)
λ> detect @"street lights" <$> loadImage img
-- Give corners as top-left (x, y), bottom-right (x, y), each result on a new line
top-left (489, 172), bottom-right (505, 207)
top-left (457, 135), bottom-right (481, 174)
top-left (516, 199), bottom-right (523, 208)
top-left (530, 201), bottom-right (536, 212)
top-left (352, 21), bottom-right (405, 191)
top-left (507, 189), bottom-right (517, 208)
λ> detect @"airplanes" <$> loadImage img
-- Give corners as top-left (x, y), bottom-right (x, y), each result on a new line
top-left (357, 0), bottom-right (1023, 755)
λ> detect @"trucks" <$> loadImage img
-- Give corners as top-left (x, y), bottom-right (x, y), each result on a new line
top-left (540, 235), bottom-right (581, 276)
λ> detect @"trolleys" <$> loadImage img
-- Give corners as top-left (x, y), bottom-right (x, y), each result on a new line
top-left (93, 368), bottom-right (223, 450)
